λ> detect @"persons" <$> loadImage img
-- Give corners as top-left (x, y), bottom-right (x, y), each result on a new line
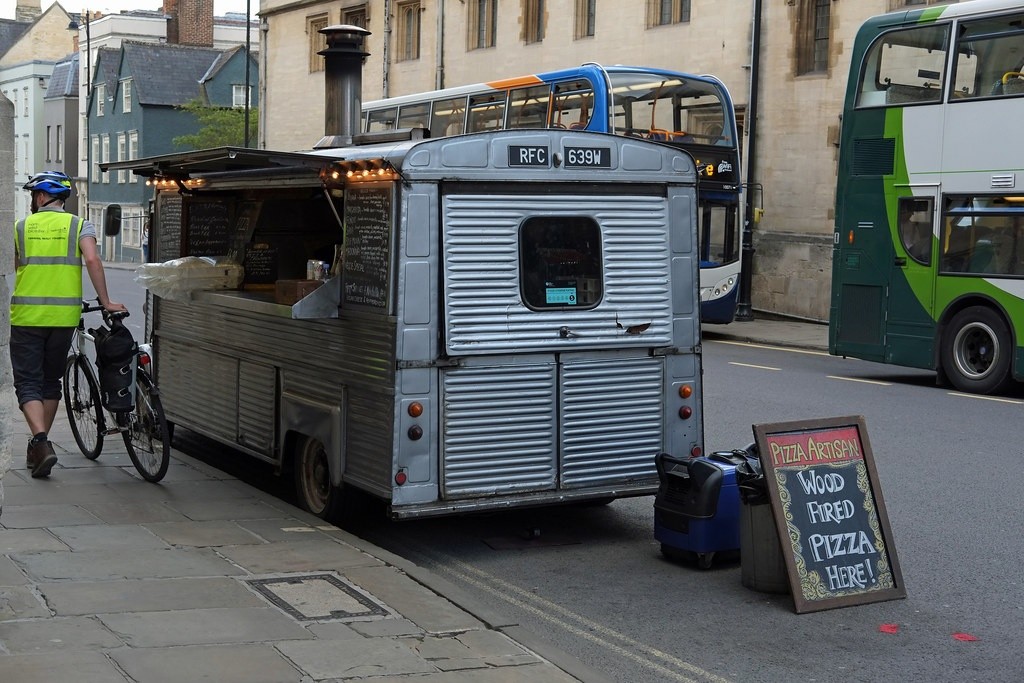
top-left (10, 171), bottom-right (127, 477)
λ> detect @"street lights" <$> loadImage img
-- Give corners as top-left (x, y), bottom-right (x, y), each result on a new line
top-left (64, 9), bottom-right (90, 220)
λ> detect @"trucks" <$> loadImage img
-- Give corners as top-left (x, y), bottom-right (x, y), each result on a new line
top-left (94, 24), bottom-right (707, 532)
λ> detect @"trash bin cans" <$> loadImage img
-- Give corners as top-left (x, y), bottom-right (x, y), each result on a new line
top-left (735, 462), bottom-right (792, 594)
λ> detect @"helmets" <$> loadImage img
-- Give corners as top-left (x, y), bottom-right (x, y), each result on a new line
top-left (22, 171), bottom-right (71, 200)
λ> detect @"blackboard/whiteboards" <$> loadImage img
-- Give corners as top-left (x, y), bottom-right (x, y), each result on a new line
top-left (752, 416), bottom-right (906, 612)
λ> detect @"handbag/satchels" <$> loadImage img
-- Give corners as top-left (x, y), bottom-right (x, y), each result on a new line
top-left (94, 319), bottom-right (135, 412)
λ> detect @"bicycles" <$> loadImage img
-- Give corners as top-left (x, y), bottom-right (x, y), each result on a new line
top-left (64, 297), bottom-right (170, 483)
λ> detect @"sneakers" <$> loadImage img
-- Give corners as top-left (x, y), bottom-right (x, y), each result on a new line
top-left (26, 439), bottom-right (35, 468)
top-left (32, 441), bottom-right (57, 477)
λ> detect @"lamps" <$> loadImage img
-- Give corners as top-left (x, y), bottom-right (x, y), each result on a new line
top-left (345, 163), bottom-right (359, 178)
top-left (146, 176), bottom-right (205, 186)
top-left (377, 161), bottom-right (389, 175)
top-left (361, 161), bottom-right (374, 177)
top-left (329, 164), bottom-right (342, 179)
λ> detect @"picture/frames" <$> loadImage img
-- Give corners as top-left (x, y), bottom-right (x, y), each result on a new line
top-left (752, 414), bottom-right (907, 616)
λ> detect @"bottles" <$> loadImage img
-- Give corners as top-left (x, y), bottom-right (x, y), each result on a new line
top-left (322, 264), bottom-right (333, 284)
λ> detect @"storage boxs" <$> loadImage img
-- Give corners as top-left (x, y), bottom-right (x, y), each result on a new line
top-left (145, 267), bottom-right (239, 289)
top-left (275, 280), bottom-right (323, 305)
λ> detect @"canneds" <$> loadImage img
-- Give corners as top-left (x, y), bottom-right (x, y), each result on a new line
top-left (306, 259), bottom-right (326, 280)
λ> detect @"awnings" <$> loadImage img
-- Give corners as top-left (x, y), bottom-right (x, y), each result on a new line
top-left (94, 145), bottom-right (345, 233)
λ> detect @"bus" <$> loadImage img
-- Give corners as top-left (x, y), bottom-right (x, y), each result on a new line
top-left (360, 62), bottom-right (763, 325)
top-left (827, 0), bottom-right (1024, 397)
